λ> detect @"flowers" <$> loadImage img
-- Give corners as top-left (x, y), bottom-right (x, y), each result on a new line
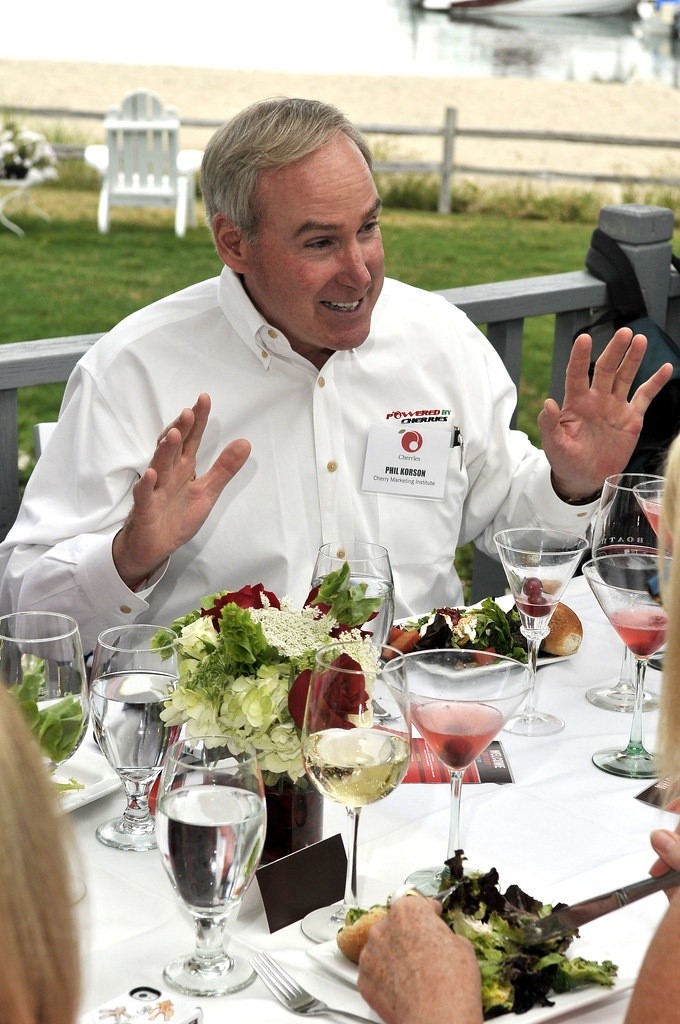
top-left (0, 122), bottom-right (59, 180)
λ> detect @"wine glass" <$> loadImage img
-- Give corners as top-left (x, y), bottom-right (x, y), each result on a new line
top-left (301, 645), bottom-right (412, 944)
top-left (383, 648), bottom-right (534, 902)
top-left (581, 555), bottom-right (672, 779)
top-left (585, 472), bottom-right (666, 713)
top-left (154, 735), bottom-right (266, 996)
top-left (492, 528), bottom-right (588, 735)
top-left (90, 624), bottom-right (181, 852)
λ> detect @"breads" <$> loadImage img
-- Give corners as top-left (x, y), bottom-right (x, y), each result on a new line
top-left (338, 908), bottom-right (387, 963)
top-left (538, 600), bottom-right (584, 655)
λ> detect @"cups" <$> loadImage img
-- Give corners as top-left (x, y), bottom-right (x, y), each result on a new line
top-left (0, 612), bottom-right (90, 778)
top-left (306, 541), bottom-right (395, 645)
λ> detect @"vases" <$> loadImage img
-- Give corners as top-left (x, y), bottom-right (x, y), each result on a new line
top-left (5, 164), bottom-right (27, 179)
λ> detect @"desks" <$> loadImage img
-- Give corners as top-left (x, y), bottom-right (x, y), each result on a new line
top-left (0, 181), bottom-right (51, 237)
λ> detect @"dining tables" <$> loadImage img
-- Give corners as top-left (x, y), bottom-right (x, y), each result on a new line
top-left (35, 573), bottom-right (680, 1024)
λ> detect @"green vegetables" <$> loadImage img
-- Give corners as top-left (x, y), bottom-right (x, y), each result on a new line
top-left (347, 847), bottom-right (620, 1018)
top-left (397, 598), bottom-right (544, 663)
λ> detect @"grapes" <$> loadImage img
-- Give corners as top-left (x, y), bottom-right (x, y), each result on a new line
top-left (523, 577), bottom-right (547, 605)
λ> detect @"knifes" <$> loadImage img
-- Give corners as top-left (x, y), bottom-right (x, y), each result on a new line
top-left (498, 868), bottom-right (680, 944)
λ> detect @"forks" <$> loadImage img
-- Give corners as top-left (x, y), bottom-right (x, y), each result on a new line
top-left (248, 952), bottom-right (365, 1024)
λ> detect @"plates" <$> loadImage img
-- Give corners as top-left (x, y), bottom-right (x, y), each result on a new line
top-left (394, 594), bottom-right (575, 676)
top-left (34, 694), bottom-right (123, 818)
top-left (305, 933), bottom-right (639, 1024)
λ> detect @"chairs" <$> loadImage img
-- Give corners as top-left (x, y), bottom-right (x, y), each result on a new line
top-left (83, 92), bottom-right (204, 237)
top-left (35, 422), bottom-right (56, 456)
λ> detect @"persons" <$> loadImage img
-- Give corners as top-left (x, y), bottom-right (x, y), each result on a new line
top-left (0, 98), bottom-right (674, 687)
top-left (356, 425), bottom-right (680, 1024)
top-left (0, 680), bottom-right (84, 1024)
